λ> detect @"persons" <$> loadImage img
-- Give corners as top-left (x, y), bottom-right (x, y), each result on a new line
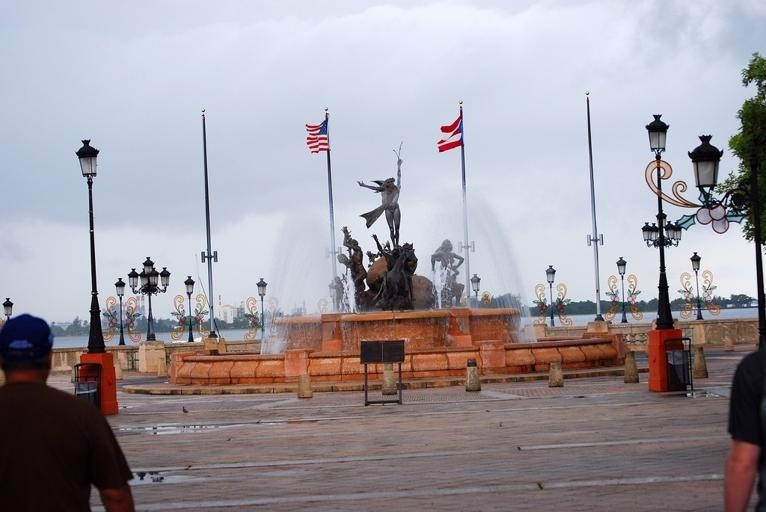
top-left (329, 158), bottom-right (465, 314)
top-left (0, 314), bottom-right (136, 512)
top-left (723, 346), bottom-right (766, 512)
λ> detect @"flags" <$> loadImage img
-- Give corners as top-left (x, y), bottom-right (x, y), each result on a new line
top-left (305, 118), bottom-right (329, 153)
top-left (437, 113), bottom-right (464, 152)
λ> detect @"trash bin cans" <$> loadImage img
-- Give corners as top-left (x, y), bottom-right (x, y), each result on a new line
top-left (662, 336), bottom-right (694, 396)
top-left (72, 362), bottom-right (103, 404)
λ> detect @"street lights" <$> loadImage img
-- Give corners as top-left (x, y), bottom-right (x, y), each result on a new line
top-left (184, 276), bottom-right (195, 341)
top-left (545, 266), bottom-right (556, 326)
top-left (643, 113), bottom-right (675, 330)
top-left (471, 273), bottom-right (481, 299)
top-left (128, 257), bottom-right (170, 341)
top-left (688, 135), bottom-right (765, 353)
top-left (690, 252), bottom-right (703, 319)
top-left (257, 279), bottom-right (267, 338)
top-left (75, 139), bottom-right (106, 353)
top-left (3, 298), bottom-right (13, 321)
top-left (617, 257), bottom-right (626, 323)
top-left (114, 278), bottom-right (125, 344)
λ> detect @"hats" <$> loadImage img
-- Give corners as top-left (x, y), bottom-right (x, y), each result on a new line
top-left (0, 314), bottom-right (54, 360)
